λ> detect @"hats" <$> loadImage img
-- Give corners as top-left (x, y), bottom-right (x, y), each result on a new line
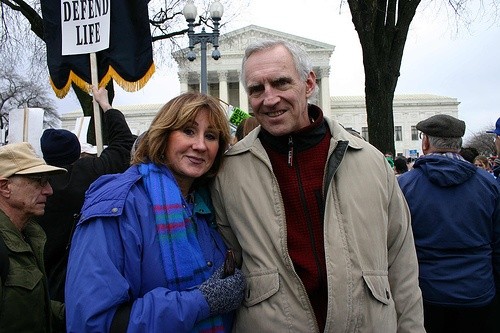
top-left (40, 129), bottom-right (81, 166)
top-left (0, 142), bottom-right (68, 179)
top-left (486, 118), bottom-right (500, 136)
top-left (459, 148), bottom-right (479, 164)
top-left (416, 114), bottom-right (465, 138)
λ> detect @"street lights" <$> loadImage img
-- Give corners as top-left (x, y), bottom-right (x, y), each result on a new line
top-left (183, 0), bottom-right (224, 95)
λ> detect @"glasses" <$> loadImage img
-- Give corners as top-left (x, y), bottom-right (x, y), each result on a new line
top-left (12, 175), bottom-right (49, 188)
top-left (492, 136), bottom-right (498, 142)
top-left (420, 132), bottom-right (428, 142)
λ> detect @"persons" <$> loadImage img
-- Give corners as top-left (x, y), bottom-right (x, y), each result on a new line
top-left (392, 111), bottom-right (500, 333)
top-left (214, 35), bottom-right (424, 332)
top-left (37, 82), bottom-right (134, 302)
top-left (62, 91), bottom-right (245, 333)
top-left (371, 118), bottom-right (500, 178)
top-left (0, 141), bottom-right (68, 332)
top-left (232, 115), bottom-right (260, 141)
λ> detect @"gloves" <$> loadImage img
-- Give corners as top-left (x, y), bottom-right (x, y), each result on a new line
top-left (197, 264), bottom-right (248, 315)
